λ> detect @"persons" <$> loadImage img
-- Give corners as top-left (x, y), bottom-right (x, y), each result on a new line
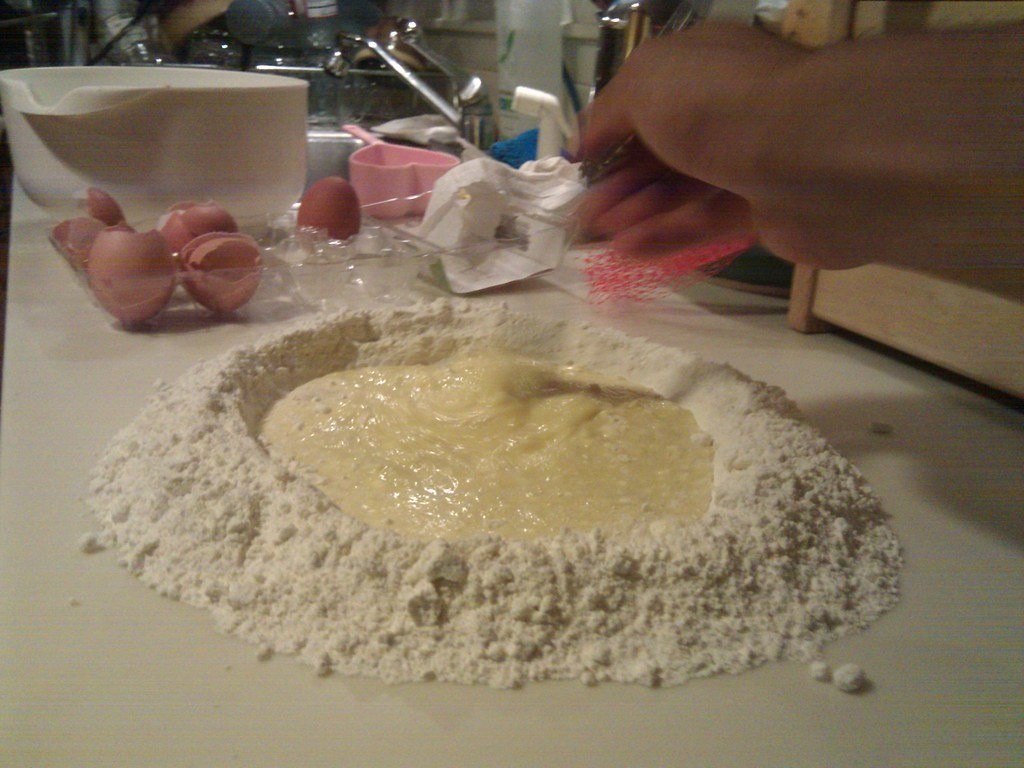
top-left (560, 19), bottom-right (1023, 278)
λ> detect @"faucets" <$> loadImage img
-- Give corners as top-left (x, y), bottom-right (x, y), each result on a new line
top-left (388, 17), bottom-right (495, 148)
top-left (326, 36), bottom-right (459, 130)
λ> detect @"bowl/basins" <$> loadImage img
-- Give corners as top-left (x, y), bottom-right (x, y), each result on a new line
top-left (0, 61), bottom-right (309, 241)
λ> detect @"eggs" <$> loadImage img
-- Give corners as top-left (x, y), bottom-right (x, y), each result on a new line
top-left (298, 177), bottom-right (361, 240)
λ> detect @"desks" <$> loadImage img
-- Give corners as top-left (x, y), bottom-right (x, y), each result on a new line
top-left (1, 117), bottom-right (1024, 768)
